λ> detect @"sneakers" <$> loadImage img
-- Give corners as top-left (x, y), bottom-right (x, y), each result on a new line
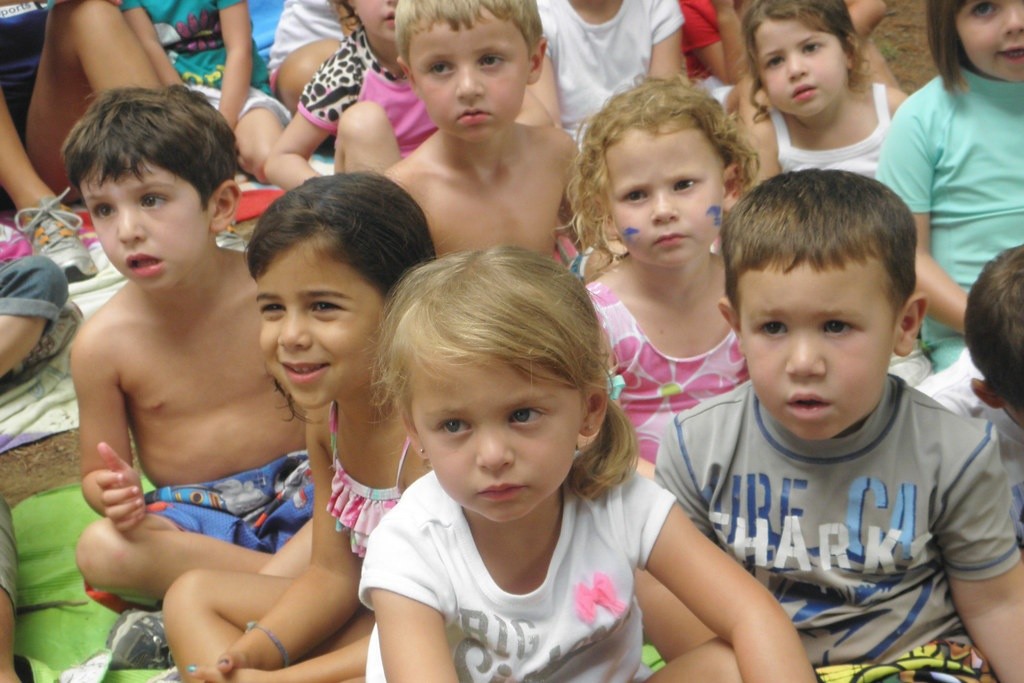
top-left (106, 599), bottom-right (175, 670)
top-left (13, 185), bottom-right (99, 280)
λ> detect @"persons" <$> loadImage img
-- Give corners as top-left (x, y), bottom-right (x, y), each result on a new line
top-left (910, 242), bottom-right (1024, 559)
top-left (373, 0), bottom-right (580, 255)
top-left (876, 0), bottom-right (1024, 370)
top-left (1, 255), bottom-right (64, 387)
top-left (262, 0), bottom-right (437, 191)
top-left (158, 173), bottom-right (439, 683)
top-left (560, 81), bottom-right (762, 486)
top-left (657, 166), bottom-right (1024, 683)
top-left (266, 2), bottom-right (348, 109)
top-left (0, 495), bottom-right (23, 683)
top-left (676, 0), bottom-right (887, 108)
top-left (526, 0), bottom-right (686, 153)
top-left (357, 244), bottom-right (818, 682)
top-left (56, 82), bottom-right (313, 606)
top-left (1, 0), bottom-right (166, 284)
top-left (731, 0), bottom-right (912, 192)
top-left (120, 1), bottom-right (294, 185)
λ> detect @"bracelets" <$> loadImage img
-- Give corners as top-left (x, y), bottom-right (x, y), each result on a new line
top-left (247, 620), bottom-right (291, 668)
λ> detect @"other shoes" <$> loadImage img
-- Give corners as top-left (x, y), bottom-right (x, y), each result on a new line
top-left (0, 302), bottom-right (84, 381)
